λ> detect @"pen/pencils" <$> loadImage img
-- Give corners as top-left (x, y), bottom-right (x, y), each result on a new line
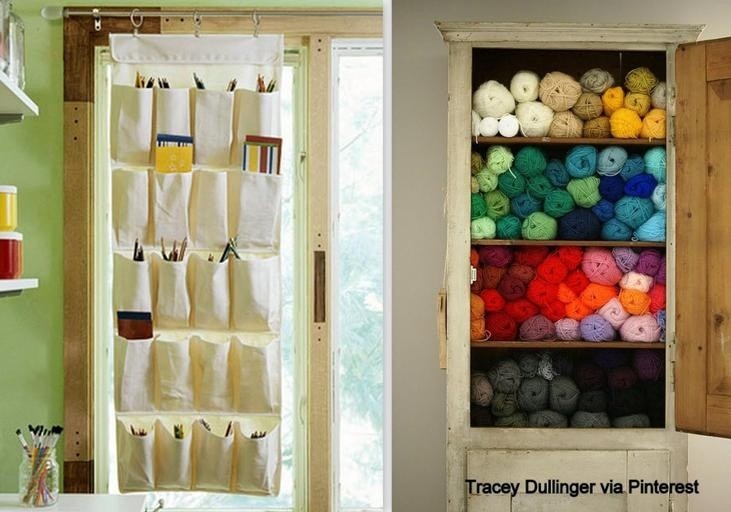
top-left (258, 74), bottom-right (278, 92)
top-left (226, 421), bottom-right (233, 437)
top-left (209, 254), bottom-right (214, 261)
top-left (130, 424), bottom-right (148, 435)
top-left (135, 72), bottom-right (155, 88)
top-left (133, 238), bottom-right (144, 261)
top-left (161, 236), bottom-right (188, 261)
top-left (220, 240), bottom-right (231, 262)
top-left (194, 74), bottom-right (204, 89)
top-left (174, 424), bottom-right (184, 438)
top-left (251, 431), bottom-right (267, 439)
top-left (228, 79), bottom-right (239, 92)
top-left (202, 418), bottom-right (211, 431)
top-left (230, 235), bottom-right (240, 259)
top-left (158, 77), bottom-right (170, 88)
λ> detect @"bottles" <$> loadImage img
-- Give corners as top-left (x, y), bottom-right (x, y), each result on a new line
top-left (18, 451), bottom-right (60, 507)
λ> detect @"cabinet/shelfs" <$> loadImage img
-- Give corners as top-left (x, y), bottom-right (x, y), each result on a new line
top-left (0, 68), bottom-right (39, 294)
top-left (434, 21), bottom-right (731, 512)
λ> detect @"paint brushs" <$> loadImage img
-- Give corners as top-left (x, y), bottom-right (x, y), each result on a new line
top-left (15, 424), bottom-right (63, 507)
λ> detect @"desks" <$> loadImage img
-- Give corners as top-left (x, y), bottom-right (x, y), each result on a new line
top-left (0, 492), bottom-right (148, 512)
